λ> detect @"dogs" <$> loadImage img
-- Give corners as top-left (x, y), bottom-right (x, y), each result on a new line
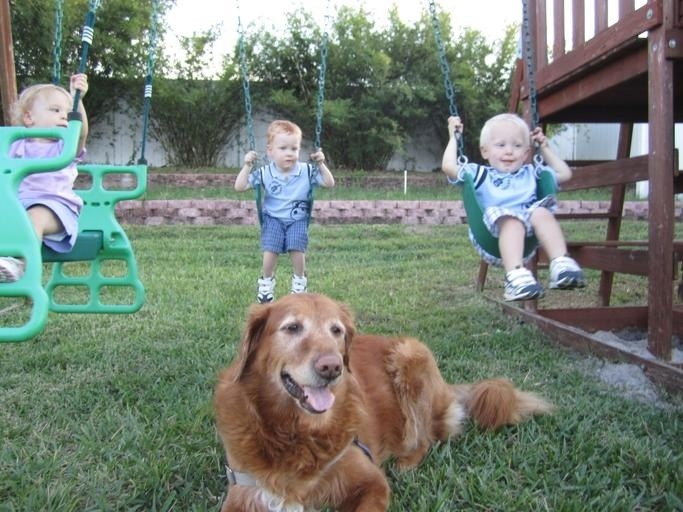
top-left (210, 292), bottom-right (557, 512)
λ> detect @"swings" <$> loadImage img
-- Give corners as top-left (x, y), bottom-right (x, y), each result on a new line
top-left (234, 0), bottom-right (333, 231)
top-left (0, 0), bottom-right (160, 342)
top-left (428, 0), bottom-right (558, 256)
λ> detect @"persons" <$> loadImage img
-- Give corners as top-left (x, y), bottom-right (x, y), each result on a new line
top-left (441, 111), bottom-right (588, 303)
top-left (233, 118), bottom-right (338, 303)
top-left (0, 73), bottom-right (91, 284)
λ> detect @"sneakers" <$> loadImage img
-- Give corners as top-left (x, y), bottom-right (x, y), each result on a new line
top-left (1, 256), bottom-right (24, 283)
top-left (547, 253), bottom-right (588, 291)
top-left (256, 277), bottom-right (277, 304)
top-left (291, 272), bottom-right (308, 295)
top-left (502, 265), bottom-right (546, 303)
